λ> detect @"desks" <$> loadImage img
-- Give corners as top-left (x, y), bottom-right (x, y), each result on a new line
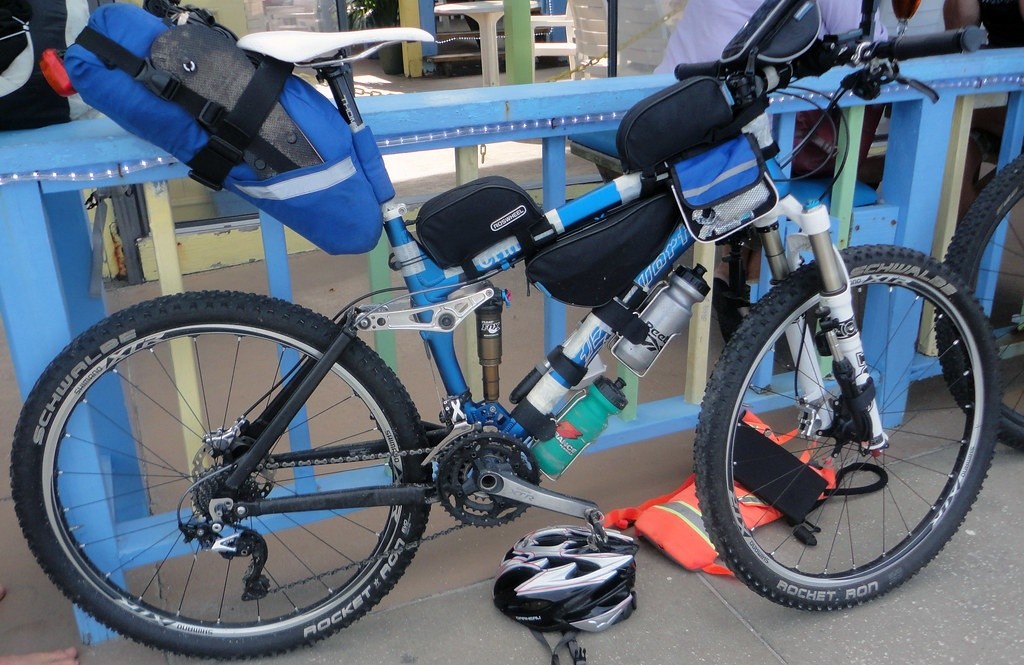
top-left (434, 0), bottom-right (538, 86)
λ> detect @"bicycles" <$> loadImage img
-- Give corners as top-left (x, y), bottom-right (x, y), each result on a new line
top-left (928, 149), bottom-right (1023, 454)
top-left (7, 0), bottom-right (1008, 662)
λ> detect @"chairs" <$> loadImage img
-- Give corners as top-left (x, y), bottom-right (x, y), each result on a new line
top-left (531, 0), bottom-right (669, 81)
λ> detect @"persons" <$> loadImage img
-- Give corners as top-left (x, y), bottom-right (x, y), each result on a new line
top-left (645, 0), bottom-right (1024, 345)
top-left (0, 0), bottom-right (108, 132)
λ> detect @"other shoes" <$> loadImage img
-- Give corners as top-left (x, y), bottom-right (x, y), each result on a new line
top-left (713, 274), bottom-right (744, 343)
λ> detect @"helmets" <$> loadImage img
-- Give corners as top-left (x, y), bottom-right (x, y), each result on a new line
top-left (492, 524), bottom-right (640, 632)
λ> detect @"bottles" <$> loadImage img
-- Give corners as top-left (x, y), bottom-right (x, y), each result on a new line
top-left (532, 375), bottom-right (629, 478)
top-left (616, 263), bottom-right (711, 370)
